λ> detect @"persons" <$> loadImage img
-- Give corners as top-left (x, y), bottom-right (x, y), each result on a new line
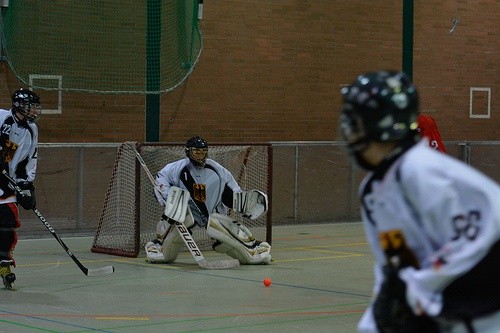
top-left (145, 136), bottom-right (272, 265)
top-left (339, 70), bottom-right (500, 333)
top-left (0, 90), bottom-right (41, 291)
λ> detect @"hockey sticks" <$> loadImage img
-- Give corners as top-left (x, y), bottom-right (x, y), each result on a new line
top-left (2, 170), bottom-right (114, 277)
top-left (131, 145), bottom-right (240, 270)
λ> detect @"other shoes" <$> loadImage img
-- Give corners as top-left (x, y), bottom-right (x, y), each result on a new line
top-left (146, 237), bottom-right (165, 264)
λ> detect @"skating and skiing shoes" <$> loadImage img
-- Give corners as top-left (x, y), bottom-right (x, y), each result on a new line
top-left (0, 264), bottom-right (17, 290)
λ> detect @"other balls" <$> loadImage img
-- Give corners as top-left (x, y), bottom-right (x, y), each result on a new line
top-left (264, 277), bottom-right (271, 287)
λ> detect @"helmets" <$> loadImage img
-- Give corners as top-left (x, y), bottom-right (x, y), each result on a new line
top-left (13, 88), bottom-right (41, 124)
top-left (341, 71), bottom-right (419, 146)
top-left (185, 136), bottom-right (209, 165)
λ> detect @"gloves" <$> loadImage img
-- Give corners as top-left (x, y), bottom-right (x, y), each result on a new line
top-left (16, 181), bottom-right (35, 209)
